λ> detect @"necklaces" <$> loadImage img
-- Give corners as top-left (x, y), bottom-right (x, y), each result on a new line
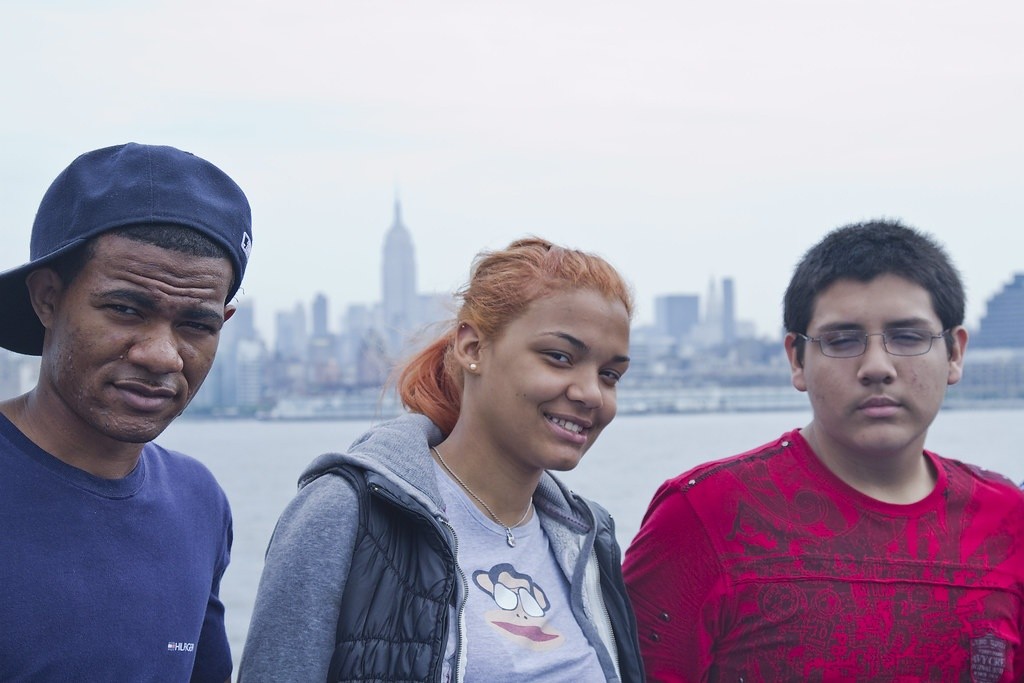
top-left (435, 448), bottom-right (533, 546)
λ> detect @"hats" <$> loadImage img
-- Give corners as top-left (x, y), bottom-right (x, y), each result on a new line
top-left (1, 142), bottom-right (255, 356)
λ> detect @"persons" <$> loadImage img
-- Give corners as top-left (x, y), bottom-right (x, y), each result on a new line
top-left (238, 235), bottom-right (621, 683)
top-left (618, 220), bottom-right (1024, 683)
top-left (0, 143), bottom-right (252, 683)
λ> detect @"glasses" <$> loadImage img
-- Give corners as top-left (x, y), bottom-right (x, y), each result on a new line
top-left (795, 328), bottom-right (949, 359)
